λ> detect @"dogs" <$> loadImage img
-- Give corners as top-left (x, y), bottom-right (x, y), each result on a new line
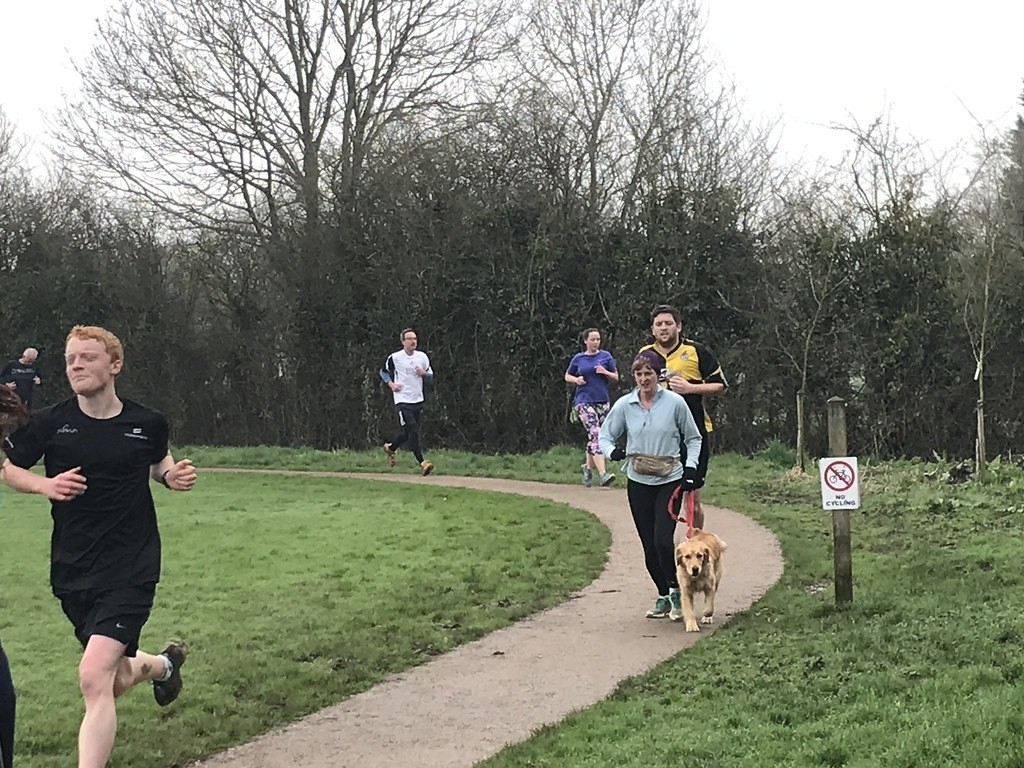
top-left (674, 528), bottom-right (727, 632)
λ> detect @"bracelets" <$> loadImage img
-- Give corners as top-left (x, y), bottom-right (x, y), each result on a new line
top-left (161, 471), bottom-right (172, 490)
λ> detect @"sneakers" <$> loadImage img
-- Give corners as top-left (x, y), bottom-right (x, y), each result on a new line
top-left (646, 598), bottom-right (671, 618)
top-left (152, 638), bottom-right (188, 706)
top-left (599, 472), bottom-right (616, 486)
top-left (668, 593), bottom-right (683, 622)
top-left (581, 464), bottom-right (593, 487)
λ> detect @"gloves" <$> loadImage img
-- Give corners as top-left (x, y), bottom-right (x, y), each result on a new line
top-left (611, 449), bottom-right (626, 461)
top-left (681, 467), bottom-right (696, 492)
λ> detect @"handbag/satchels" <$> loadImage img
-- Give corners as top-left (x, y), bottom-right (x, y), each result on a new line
top-left (627, 456), bottom-right (681, 477)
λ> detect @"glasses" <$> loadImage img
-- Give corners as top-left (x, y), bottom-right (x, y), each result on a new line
top-left (403, 337), bottom-right (418, 341)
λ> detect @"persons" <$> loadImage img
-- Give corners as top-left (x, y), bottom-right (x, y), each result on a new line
top-left (639, 304), bottom-right (728, 529)
top-left (563, 328), bottom-right (617, 489)
top-left (0, 348), bottom-right (44, 415)
top-left (0, 325), bottom-right (197, 767)
top-left (598, 351), bottom-right (703, 623)
top-left (378, 328), bottom-right (434, 478)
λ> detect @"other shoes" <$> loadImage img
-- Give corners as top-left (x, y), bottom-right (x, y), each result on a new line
top-left (421, 460), bottom-right (433, 476)
top-left (384, 443), bottom-right (397, 466)
